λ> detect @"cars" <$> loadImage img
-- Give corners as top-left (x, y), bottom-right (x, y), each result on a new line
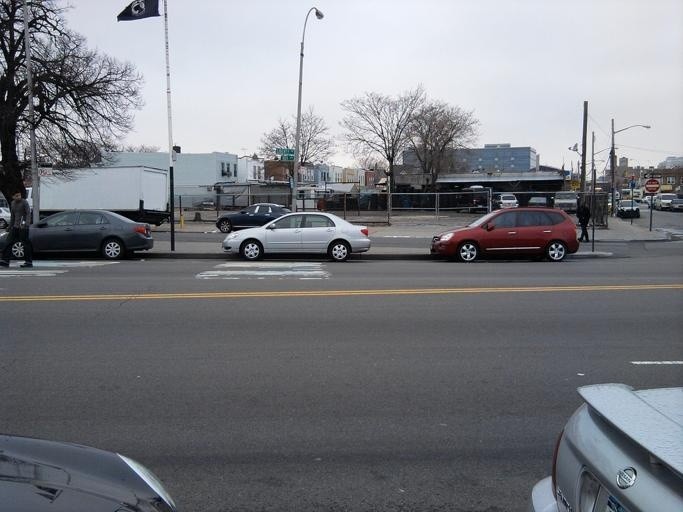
top-left (526, 378), bottom-right (682, 512)
top-left (0, 428), bottom-right (183, 512)
top-left (220, 209), bottom-right (374, 263)
top-left (0, 207), bottom-right (155, 261)
top-left (1, 207), bottom-right (12, 230)
top-left (429, 205), bottom-right (582, 264)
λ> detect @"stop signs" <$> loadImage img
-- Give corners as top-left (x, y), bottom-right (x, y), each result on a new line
top-left (644, 179), bottom-right (661, 193)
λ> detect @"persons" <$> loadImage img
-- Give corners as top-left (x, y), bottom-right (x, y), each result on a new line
top-left (0, 190), bottom-right (34, 268)
top-left (575, 197), bottom-right (591, 242)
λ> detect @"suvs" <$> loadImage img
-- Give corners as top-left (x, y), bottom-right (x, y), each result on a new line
top-left (213, 200), bottom-right (293, 234)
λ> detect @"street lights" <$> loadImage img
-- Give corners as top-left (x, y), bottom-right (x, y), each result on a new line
top-left (290, 4), bottom-right (325, 213)
top-left (591, 146), bottom-right (619, 187)
top-left (610, 125), bottom-right (651, 219)
top-left (585, 160), bottom-right (605, 167)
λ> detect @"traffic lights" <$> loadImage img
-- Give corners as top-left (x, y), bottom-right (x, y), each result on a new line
top-left (280, 155), bottom-right (294, 161)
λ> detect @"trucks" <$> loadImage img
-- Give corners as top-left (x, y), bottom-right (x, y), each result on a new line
top-left (21, 164), bottom-right (170, 224)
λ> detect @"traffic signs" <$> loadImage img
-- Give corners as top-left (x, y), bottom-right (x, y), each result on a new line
top-left (276, 148), bottom-right (294, 155)
top-left (643, 173), bottom-right (663, 178)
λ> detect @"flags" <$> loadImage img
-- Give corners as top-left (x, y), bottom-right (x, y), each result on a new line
top-left (117, 0), bottom-right (161, 22)
